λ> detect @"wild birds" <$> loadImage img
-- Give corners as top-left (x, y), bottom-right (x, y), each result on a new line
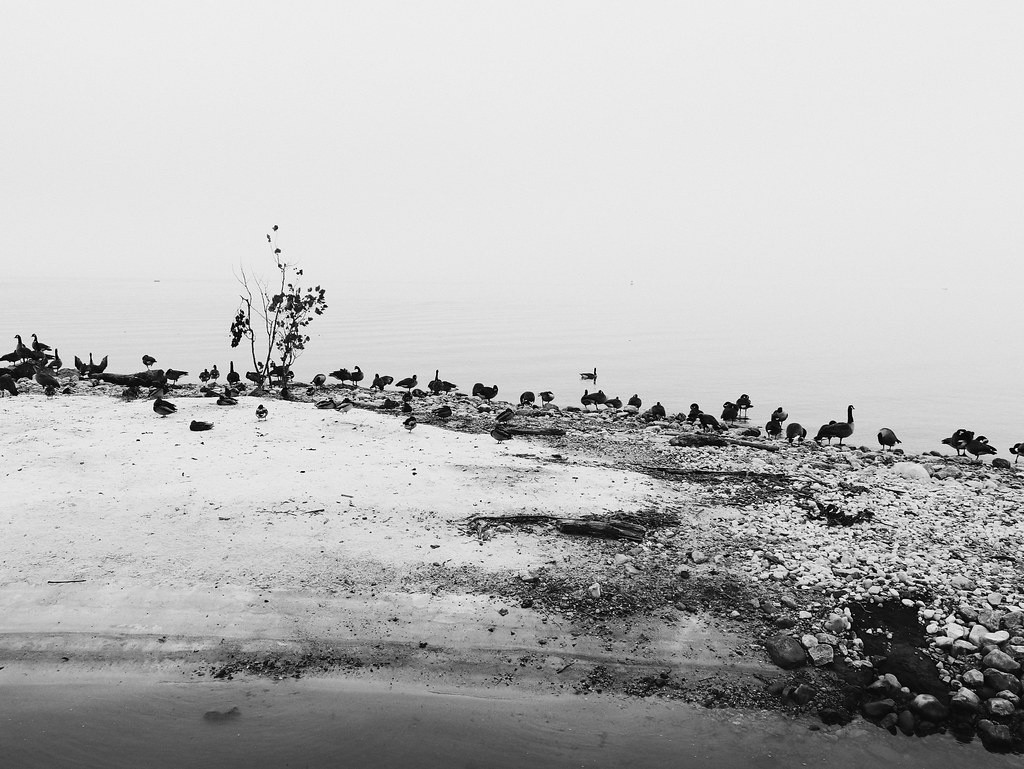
top-left (0, 332), bottom-right (1024, 469)
